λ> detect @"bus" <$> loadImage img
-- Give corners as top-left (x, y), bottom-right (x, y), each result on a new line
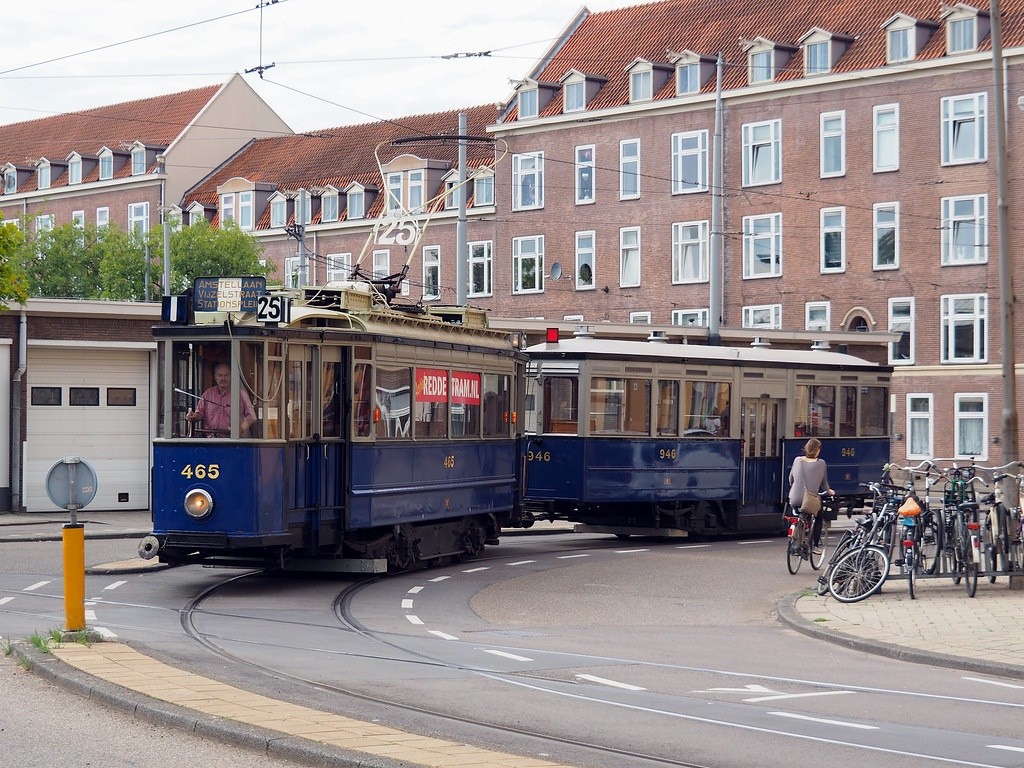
top-left (138, 134), bottom-right (896, 580)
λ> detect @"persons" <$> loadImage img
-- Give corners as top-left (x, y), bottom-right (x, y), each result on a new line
top-left (707, 401), bottom-right (729, 435)
top-left (788, 438), bottom-right (836, 555)
top-left (184, 363), bottom-right (257, 437)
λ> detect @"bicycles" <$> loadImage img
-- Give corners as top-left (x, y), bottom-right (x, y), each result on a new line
top-left (784, 490), bottom-right (837, 574)
top-left (817, 456), bottom-right (1024, 603)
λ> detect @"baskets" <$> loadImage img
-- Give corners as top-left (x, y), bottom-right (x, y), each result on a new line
top-left (822, 502), bottom-right (838, 520)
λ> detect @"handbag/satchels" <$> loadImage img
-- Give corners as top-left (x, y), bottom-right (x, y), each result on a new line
top-left (801, 489), bottom-right (821, 517)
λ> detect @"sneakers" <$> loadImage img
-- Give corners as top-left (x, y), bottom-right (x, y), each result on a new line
top-left (811, 546), bottom-right (822, 554)
